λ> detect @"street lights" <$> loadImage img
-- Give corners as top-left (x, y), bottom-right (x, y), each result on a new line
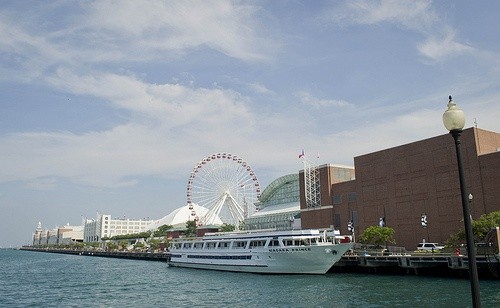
top-left (442, 95), bottom-right (481, 308)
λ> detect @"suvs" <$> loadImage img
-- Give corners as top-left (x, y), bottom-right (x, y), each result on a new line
top-left (417, 243), bottom-right (445, 251)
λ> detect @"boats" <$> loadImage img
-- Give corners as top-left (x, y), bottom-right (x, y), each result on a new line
top-left (163, 228), bottom-right (353, 275)
